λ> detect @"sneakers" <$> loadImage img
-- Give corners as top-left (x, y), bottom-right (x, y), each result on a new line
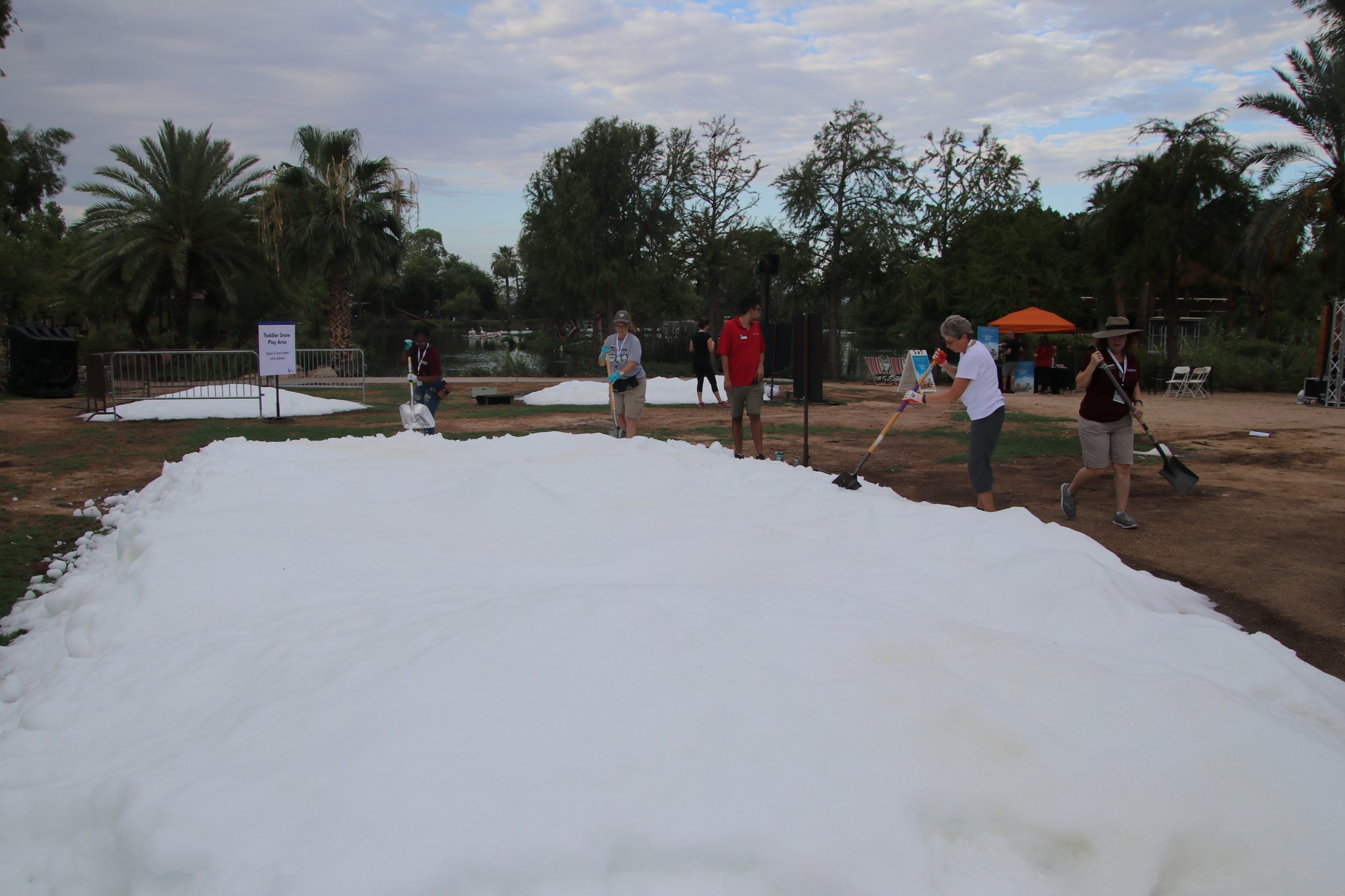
top-left (717, 400), bottom-right (730, 408)
top-left (1112, 510), bottom-right (1137, 528)
top-left (698, 402), bottom-right (707, 408)
top-left (1060, 483), bottom-right (1078, 519)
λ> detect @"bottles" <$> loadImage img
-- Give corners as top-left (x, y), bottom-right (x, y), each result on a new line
top-left (776, 451), bottom-right (784, 461)
top-left (792, 456), bottom-right (800, 468)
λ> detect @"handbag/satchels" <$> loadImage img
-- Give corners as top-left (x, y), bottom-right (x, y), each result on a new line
top-left (612, 378), bottom-right (627, 393)
top-left (434, 380), bottom-right (452, 401)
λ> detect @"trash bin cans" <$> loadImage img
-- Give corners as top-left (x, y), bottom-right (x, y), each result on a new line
top-left (7, 324), bottom-right (82, 398)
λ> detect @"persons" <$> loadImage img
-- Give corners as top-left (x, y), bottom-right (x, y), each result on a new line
top-left (1061, 317), bottom-right (1143, 528)
top-left (1033, 336), bottom-right (1056, 394)
top-left (724, 316), bottom-right (732, 325)
top-left (716, 294), bottom-right (766, 460)
top-left (599, 310), bottom-right (646, 438)
top-left (400, 325), bottom-right (442, 434)
top-left (690, 318), bottom-right (730, 407)
top-left (999, 330), bottom-right (1026, 394)
top-left (901, 315), bottom-right (1005, 512)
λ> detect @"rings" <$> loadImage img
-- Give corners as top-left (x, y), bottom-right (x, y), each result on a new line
top-left (1099, 357), bottom-right (1101, 359)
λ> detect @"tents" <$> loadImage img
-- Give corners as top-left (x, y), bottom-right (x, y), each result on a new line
top-left (988, 307), bottom-right (1077, 394)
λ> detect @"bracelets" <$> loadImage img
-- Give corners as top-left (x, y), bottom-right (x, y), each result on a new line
top-left (1134, 400), bottom-right (1143, 407)
top-left (1003, 353), bottom-right (1004, 356)
top-left (923, 395), bottom-right (926, 403)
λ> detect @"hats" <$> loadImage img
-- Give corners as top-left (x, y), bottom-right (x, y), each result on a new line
top-left (614, 311), bottom-right (630, 324)
top-left (1092, 316), bottom-right (1143, 338)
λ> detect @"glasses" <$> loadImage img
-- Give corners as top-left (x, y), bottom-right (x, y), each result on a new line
top-left (724, 316), bottom-right (731, 319)
top-left (943, 337), bottom-right (958, 345)
top-left (415, 338), bottom-right (430, 345)
top-left (614, 323), bottom-right (630, 330)
top-left (755, 308), bottom-right (762, 314)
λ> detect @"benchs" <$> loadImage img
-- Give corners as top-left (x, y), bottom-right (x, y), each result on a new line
top-left (471, 386), bottom-right (514, 405)
top-left (1147, 365), bottom-right (1213, 395)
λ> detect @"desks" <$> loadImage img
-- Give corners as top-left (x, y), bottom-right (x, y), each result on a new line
top-left (998, 366), bottom-right (1068, 394)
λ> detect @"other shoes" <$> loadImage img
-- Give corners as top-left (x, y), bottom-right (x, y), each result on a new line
top-left (1037, 390), bottom-right (1041, 394)
top-left (1047, 391), bottom-right (1052, 395)
top-left (734, 453), bottom-right (744, 460)
top-left (755, 453), bottom-right (765, 461)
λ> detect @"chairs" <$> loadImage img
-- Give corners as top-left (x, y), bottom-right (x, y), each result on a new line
top-left (0, 359), bottom-right (9, 392)
top-left (1164, 366), bottom-right (1211, 398)
top-left (862, 356), bottom-right (893, 387)
top-left (886, 356), bottom-right (905, 386)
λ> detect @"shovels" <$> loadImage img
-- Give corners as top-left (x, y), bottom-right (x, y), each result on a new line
top-left (831, 350), bottom-right (944, 490)
top-left (1088, 343), bottom-right (1199, 497)
top-left (398, 341), bottom-right (436, 429)
top-left (603, 340), bottom-right (627, 439)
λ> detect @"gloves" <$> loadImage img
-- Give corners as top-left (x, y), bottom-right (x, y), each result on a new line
top-left (902, 390), bottom-right (925, 404)
top-left (407, 373), bottom-right (417, 382)
top-left (601, 345), bottom-right (611, 358)
top-left (606, 373), bottom-right (621, 384)
top-left (404, 339), bottom-right (413, 350)
top-left (932, 348), bottom-right (948, 367)
